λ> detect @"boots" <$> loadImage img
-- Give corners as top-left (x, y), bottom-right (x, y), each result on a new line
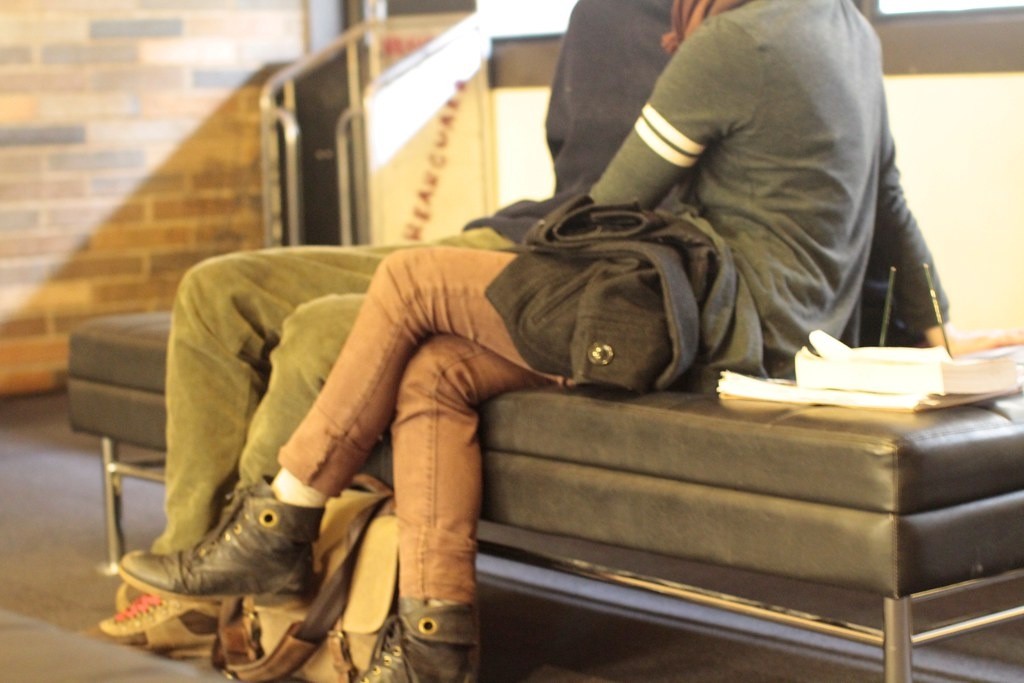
top-left (121, 474), bottom-right (325, 608)
top-left (352, 598), bottom-right (479, 682)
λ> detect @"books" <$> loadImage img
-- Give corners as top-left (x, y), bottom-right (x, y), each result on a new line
top-left (717, 329), bottom-right (1024, 413)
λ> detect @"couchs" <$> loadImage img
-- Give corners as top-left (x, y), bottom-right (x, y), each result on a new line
top-left (65, 308), bottom-right (1021, 683)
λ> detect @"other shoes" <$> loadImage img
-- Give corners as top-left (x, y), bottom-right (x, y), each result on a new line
top-left (100, 582), bottom-right (216, 644)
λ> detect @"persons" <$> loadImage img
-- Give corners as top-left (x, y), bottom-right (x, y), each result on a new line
top-left (117, 1), bottom-right (951, 683)
top-left (98, 1), bottom-right (682, 653)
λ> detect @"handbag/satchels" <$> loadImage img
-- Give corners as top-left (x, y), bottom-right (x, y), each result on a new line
top-left (212, 475), bottom-right (401, 683)
